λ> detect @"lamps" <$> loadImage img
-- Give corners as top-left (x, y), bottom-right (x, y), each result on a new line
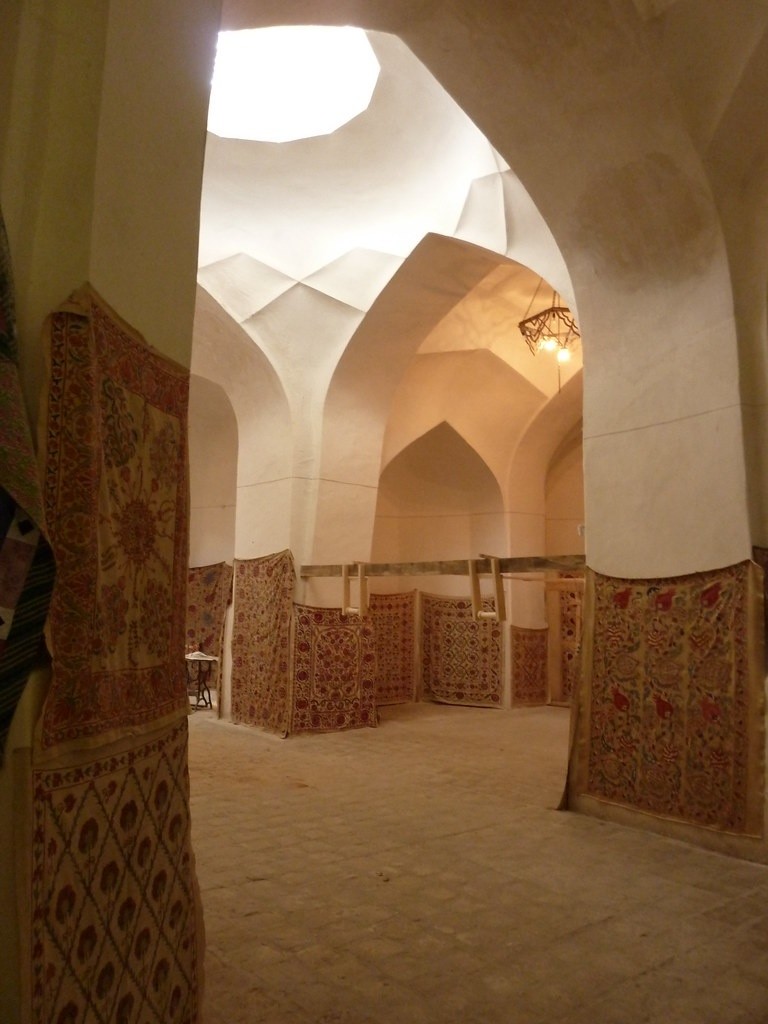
top-left (518, 276), bottom-right (582, 366)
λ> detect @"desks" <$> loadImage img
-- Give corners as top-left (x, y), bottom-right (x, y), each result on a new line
top-left (183, 655), bottom-right (218, 712)
